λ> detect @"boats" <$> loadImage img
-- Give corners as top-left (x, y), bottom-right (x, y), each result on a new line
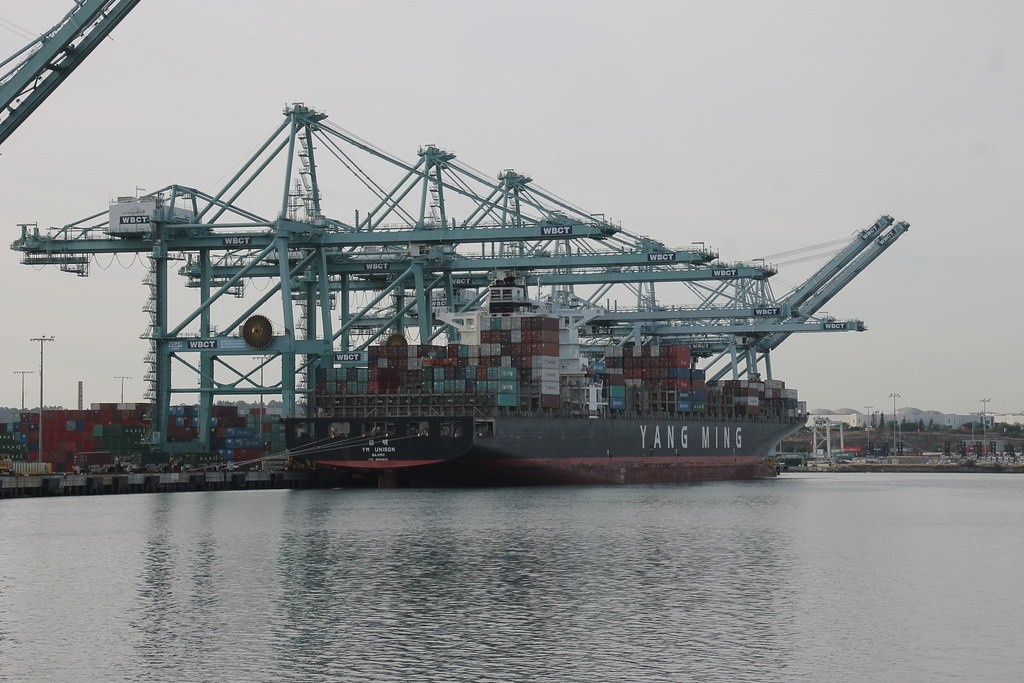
top-left (276, 295), bottom-right (810, 490)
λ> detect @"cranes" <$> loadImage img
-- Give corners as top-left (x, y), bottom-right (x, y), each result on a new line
top-left (0, 0), bottom-right (918, 467)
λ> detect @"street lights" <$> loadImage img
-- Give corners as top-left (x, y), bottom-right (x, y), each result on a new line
top-left (980, 397), bottom-right (991, 459)
top-left (11, 371), bottom-right (33, 410)
top-left (252, 357), bottom-right (273, 444)
top-left (113, 374), bottom-right (133, 402)
top-left (889, 392), bottom-right (902, 456)
top-left (864, 405), bottom-right (875, 448)
top-left (30, 335), bottom-right (56, 462)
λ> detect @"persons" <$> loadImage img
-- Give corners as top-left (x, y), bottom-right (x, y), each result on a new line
top-left (828, 461), bottom-right (832, 466)
